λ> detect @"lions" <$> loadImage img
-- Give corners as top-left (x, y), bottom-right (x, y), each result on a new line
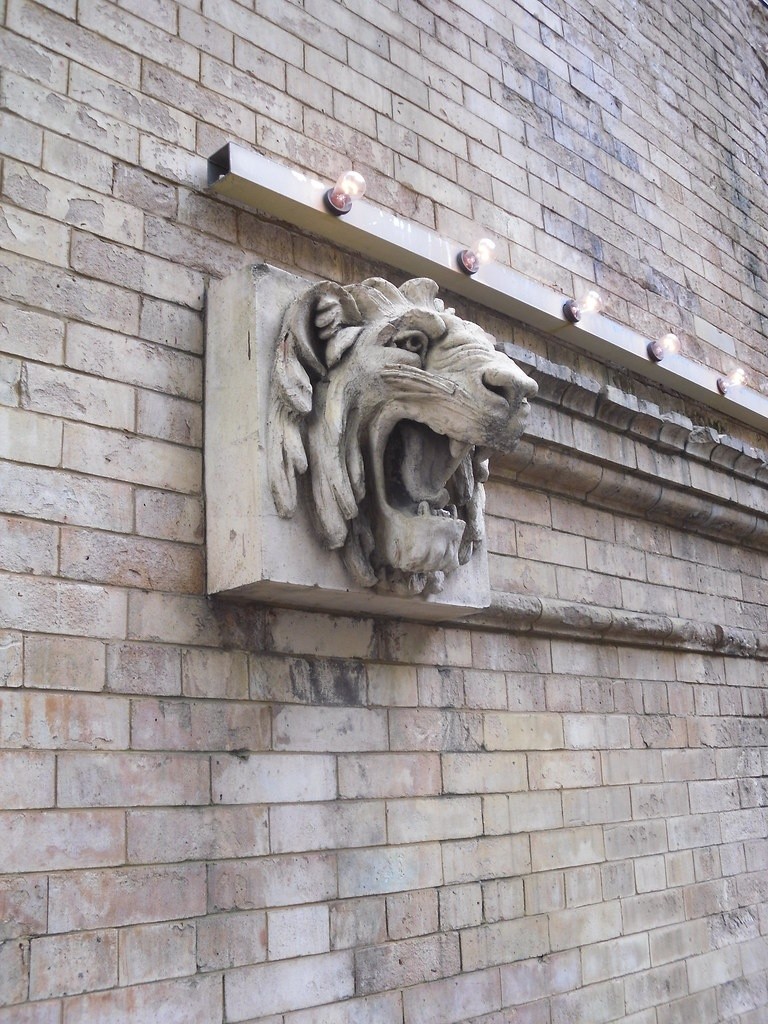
top-left (267, 278), bottom-right (537, 599)
